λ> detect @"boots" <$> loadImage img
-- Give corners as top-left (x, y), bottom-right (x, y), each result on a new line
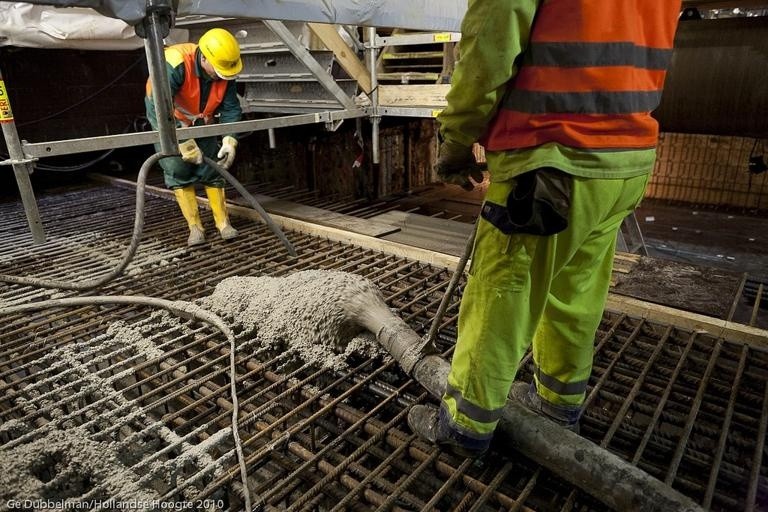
top-left (205, 185), bottom-right (239, 240)
top-left (174, 185), bottom-right (206, 246)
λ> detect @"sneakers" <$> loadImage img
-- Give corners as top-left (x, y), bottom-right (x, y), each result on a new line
top-left (407, 404), bottom-right (490, 458)
top-left (508, 381), bottom-right (581, 435)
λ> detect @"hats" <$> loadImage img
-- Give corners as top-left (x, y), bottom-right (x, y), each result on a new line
top-left (214, 68), bottom-right (239, 81)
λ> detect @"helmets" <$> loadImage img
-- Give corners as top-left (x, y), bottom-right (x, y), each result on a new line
top-left (198, 28), bottom-right (244, 76)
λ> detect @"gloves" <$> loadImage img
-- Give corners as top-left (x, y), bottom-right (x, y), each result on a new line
top-left (217, 136), bottom-right (238, 170)
top-left (434, 136), bottom-right (490, 192)
top-left (179, 139), bottom-right (203, 165)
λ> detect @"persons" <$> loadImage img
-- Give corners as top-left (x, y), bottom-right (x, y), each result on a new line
top-left (142, 26), bottom-right (245, 247)
top-left (405, 0), bottom-right (684, 459)
